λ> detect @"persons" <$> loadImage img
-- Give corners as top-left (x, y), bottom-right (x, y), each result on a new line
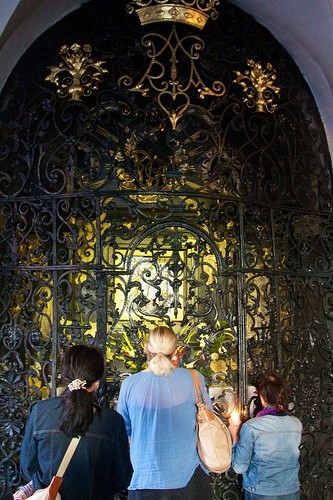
top-left (116, 326), bottom-right (214, 500)
top-left (228, 374), bottom-right (303, 500)
top-left (20, 344), bottom-right (134, 500)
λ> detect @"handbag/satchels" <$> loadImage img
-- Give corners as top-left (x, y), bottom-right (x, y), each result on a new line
top-left (193, 402), bottom-right (232, 474)
top-left (10, 476), bottom-right (61, 500)
